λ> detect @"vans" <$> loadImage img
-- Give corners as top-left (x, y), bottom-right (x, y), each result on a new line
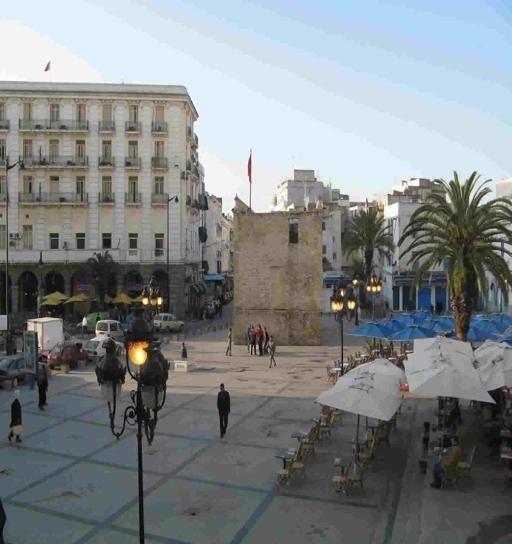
top-left (95, 319), bottom-right (124, 338)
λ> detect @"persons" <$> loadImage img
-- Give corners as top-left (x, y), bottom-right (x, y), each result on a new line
top-left (82, 312), bottom-right (89, 334)
top-left (8, 399), bottom-right (22, 442)
top-left (265, 335), bottom-right (276, 368)
top-left (37, 365), bottom-right (48, 410)
top-left (126, 312), bottom-right (133, 325)
top-left (248, 325), bottom-right (269, 356)
top-left (96, 313), bottom-right (101, 321)
top-left (430, 436), bottom-right (461, 488)
top-left (181, 343), bottom-right (187, 360)
top-left (199, 302), bottom-right (222, 320)
top-left (217, 384), bottom-right (230, 438)
top-left (226, 328), bottom-right (232, 356)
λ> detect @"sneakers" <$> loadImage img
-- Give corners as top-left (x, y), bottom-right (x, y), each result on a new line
top-left (8, 436), bottom-right (22, 443)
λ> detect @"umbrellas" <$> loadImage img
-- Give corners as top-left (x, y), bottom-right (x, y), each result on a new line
top-left (313, 311), bottom-right (512, 463)
top-left (40, 291), bottom-right (142, 305)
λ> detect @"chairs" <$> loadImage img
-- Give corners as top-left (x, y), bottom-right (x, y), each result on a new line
top-left (277, 405), bottom-right (397, 493)
top-left (433, 395), bottom-right (475, 491)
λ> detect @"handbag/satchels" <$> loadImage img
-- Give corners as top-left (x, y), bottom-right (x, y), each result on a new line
top-left (13, 425), bottom-right (24, 435)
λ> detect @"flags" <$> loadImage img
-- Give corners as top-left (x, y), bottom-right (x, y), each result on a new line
top-left (248, 154), bottom-right (251, 182)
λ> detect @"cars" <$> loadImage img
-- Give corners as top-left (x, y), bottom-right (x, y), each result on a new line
top-left (152, 313), bottom-right (186, 333)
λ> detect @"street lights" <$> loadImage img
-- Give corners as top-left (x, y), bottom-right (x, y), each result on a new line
top-left (93, 301), bottom-right (171, 544)
top-left (330, 274), bottom-right (383, 375)
top-left (166, 194), bottom-right (179, 313)
top-left (141, 274), bottom-right (163, 312)
top-left (5, 156), bottom-right (27, 331)
